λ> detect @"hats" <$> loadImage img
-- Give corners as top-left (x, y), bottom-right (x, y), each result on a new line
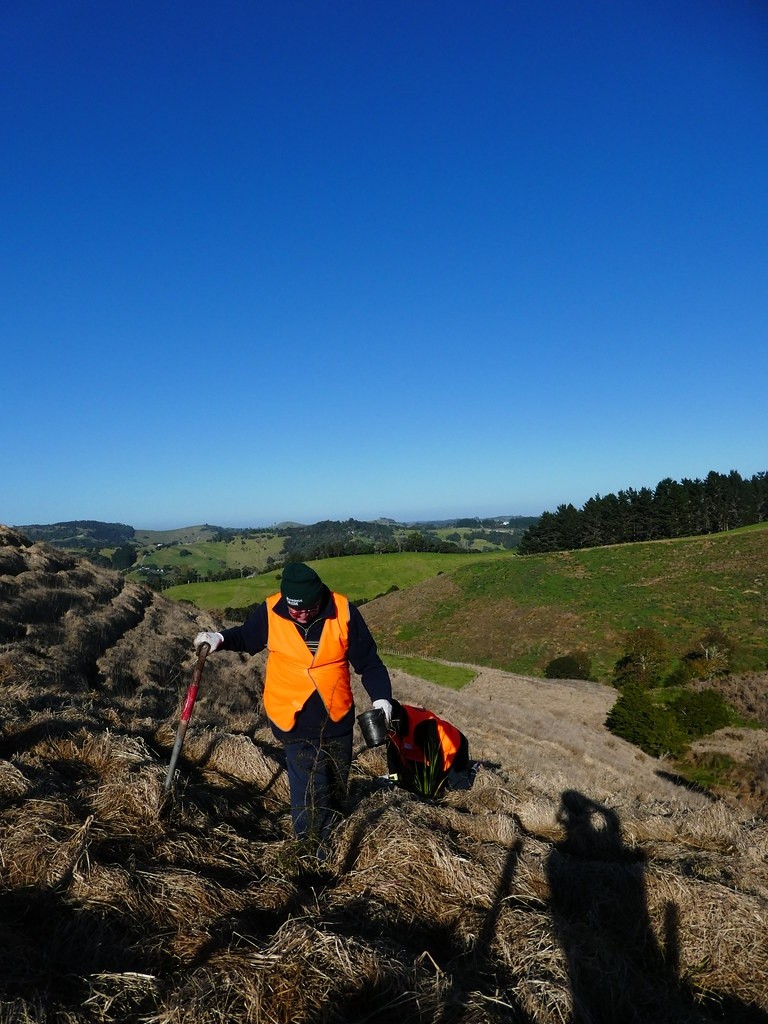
top-left (280, 562), bottom-right (323, 611)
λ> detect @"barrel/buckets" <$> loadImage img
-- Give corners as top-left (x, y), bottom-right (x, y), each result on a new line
top-left (357, 708), bottom-right (389, 747)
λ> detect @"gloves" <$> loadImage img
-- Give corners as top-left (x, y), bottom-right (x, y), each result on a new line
top-left (193, 631), bottom-right (225, 655)
top-left (373, 699), bottom-right (392, 723)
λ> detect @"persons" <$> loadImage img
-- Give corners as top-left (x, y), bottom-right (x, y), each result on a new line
top-left (193, 560), bottom-right (396, 858)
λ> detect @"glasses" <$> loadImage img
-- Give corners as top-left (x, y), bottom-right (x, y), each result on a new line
top-left (289, 608), bottom-right (319, 616)
